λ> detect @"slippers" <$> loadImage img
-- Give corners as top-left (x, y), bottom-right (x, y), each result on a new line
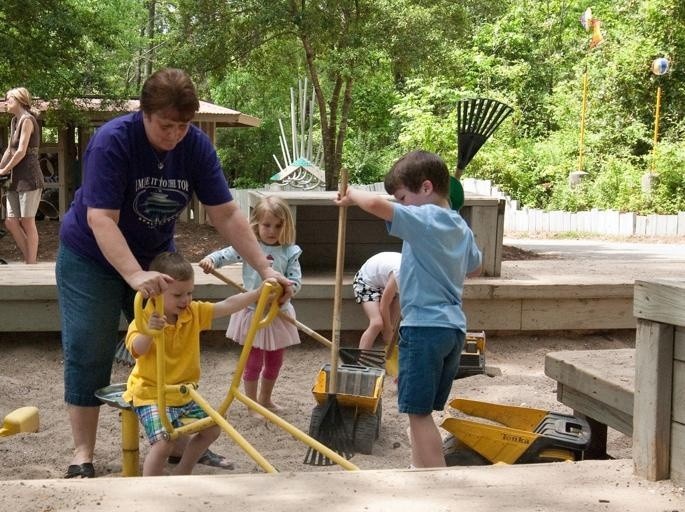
top-left (66, 461), bottom-right (96, 479)
top-left (168, 449), bottom-right (233, 468)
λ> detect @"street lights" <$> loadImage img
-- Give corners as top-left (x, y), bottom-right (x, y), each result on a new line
top-left (649, 57), bottom-right (670, 165)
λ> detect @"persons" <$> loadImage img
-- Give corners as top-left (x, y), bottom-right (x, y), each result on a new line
top-left (200, 195), bottom-right (302, 418)
top-left (121, 250), bottom-right (280, 477)
top-left (331, 148), bottom-right (484, 468)
top-left (52, 67), bottom-right (296, 479)
top-left (0, 87), bottom-right (45, 264)
top-left (353, 250), bottom-right (401, 364)
